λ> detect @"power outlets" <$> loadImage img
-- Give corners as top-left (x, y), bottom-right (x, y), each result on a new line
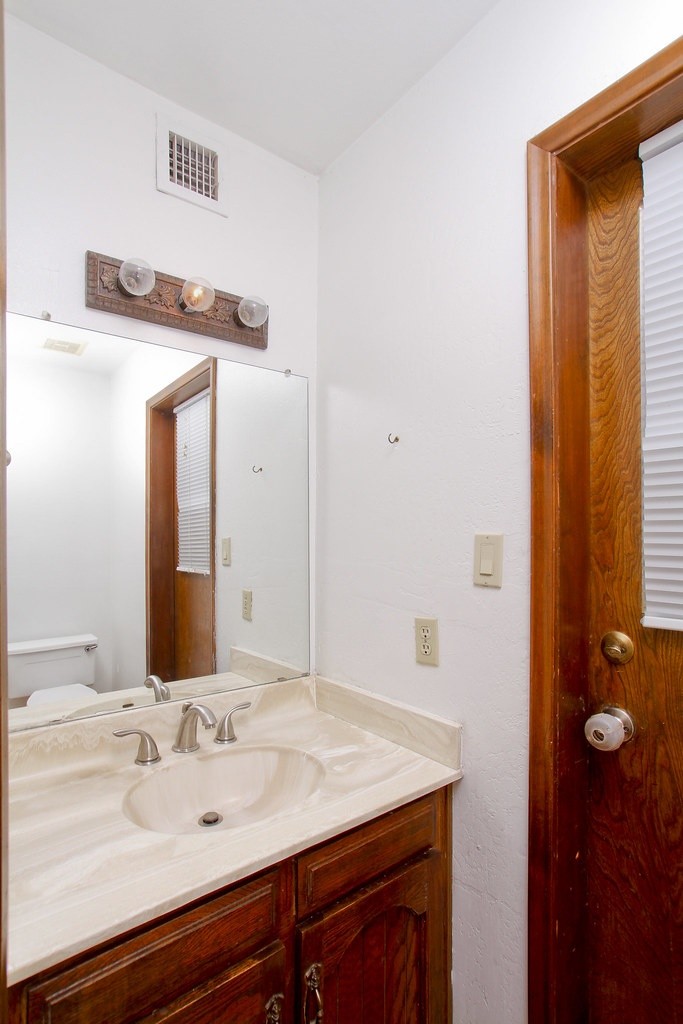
top-left (414, 617), bottom-right (439, 667)
top-left (241, 588), bottom-right (252, 622)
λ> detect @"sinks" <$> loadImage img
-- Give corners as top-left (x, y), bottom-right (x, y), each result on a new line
top-left (121, 746), bottom-right (326, 834)
top-left (63, 692), bottom-right (198, 720)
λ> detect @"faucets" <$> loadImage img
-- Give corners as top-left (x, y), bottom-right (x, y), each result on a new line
top-left (170, 701), bottom-right (219, 753)
top-left (145, 675), bottom-right (171, 703)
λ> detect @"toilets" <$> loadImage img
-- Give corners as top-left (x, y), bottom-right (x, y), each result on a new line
top-left (8, 634), bottom-right (98, 707)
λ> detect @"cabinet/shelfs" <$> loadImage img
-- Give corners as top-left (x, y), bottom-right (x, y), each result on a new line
top-left (25, 792), bottom-right (449, 1024)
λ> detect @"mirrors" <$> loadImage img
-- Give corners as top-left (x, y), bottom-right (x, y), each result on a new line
top-left (0, 310), bottom-right (311, 733)
